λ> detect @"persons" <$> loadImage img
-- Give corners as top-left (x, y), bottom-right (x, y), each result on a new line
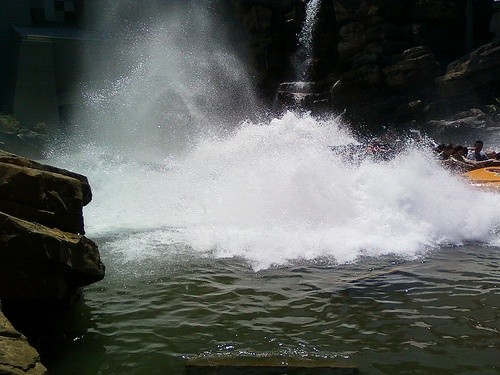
top-left (323, 137), bottom-right (500, 176)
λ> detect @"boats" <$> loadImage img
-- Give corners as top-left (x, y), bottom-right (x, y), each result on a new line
top-left (447, 160), bottom-right (500, 193)
